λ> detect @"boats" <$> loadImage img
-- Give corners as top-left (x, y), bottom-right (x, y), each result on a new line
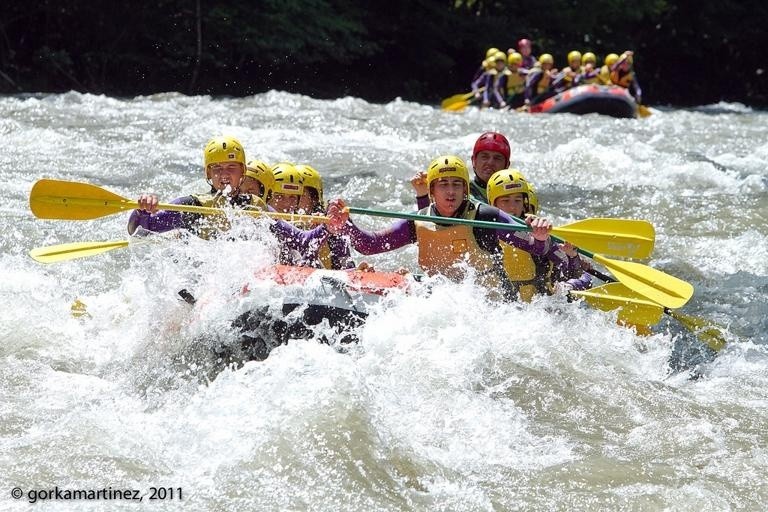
top-left (516, 82), bottom-right (642, 123)
top-left (207, 263), bottom-right (405, 372)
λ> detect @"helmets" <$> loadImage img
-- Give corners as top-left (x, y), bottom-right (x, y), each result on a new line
top-left (473, 133), bottom-right (510, 162)
top-left (486, 169), bottom-right (538, 215)
top-left (203, 137), bottom-right (247, 179)
top-left (294, 164), bottom-right (325, 204)
top-left (568, 51), bottom-right (633, 66)
top-left (244, 160), bottom-right (275, 204)
top-left (427, 155), bottom-right (470, 201)
top-left (271, 162), bottom-right (303, 209)
top-left (487, 40), bottom-right (553, 65)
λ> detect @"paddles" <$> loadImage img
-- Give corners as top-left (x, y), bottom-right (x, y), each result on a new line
top-left (29, 179), bottom-right (349, 225)
top-left (511, 215), bottom-right (726, 352)
top-left (28, 240), bottom-right (127, 263)
top-left (343, 206), bottom-right (655, 260)
top-left (441, 86), bottom-right (486, 112)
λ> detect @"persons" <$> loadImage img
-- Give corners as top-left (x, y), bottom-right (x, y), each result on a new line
top-left (129, 132), bottom-right (594, 311)
top-left (470, 38), bottom-right (642, 112)
